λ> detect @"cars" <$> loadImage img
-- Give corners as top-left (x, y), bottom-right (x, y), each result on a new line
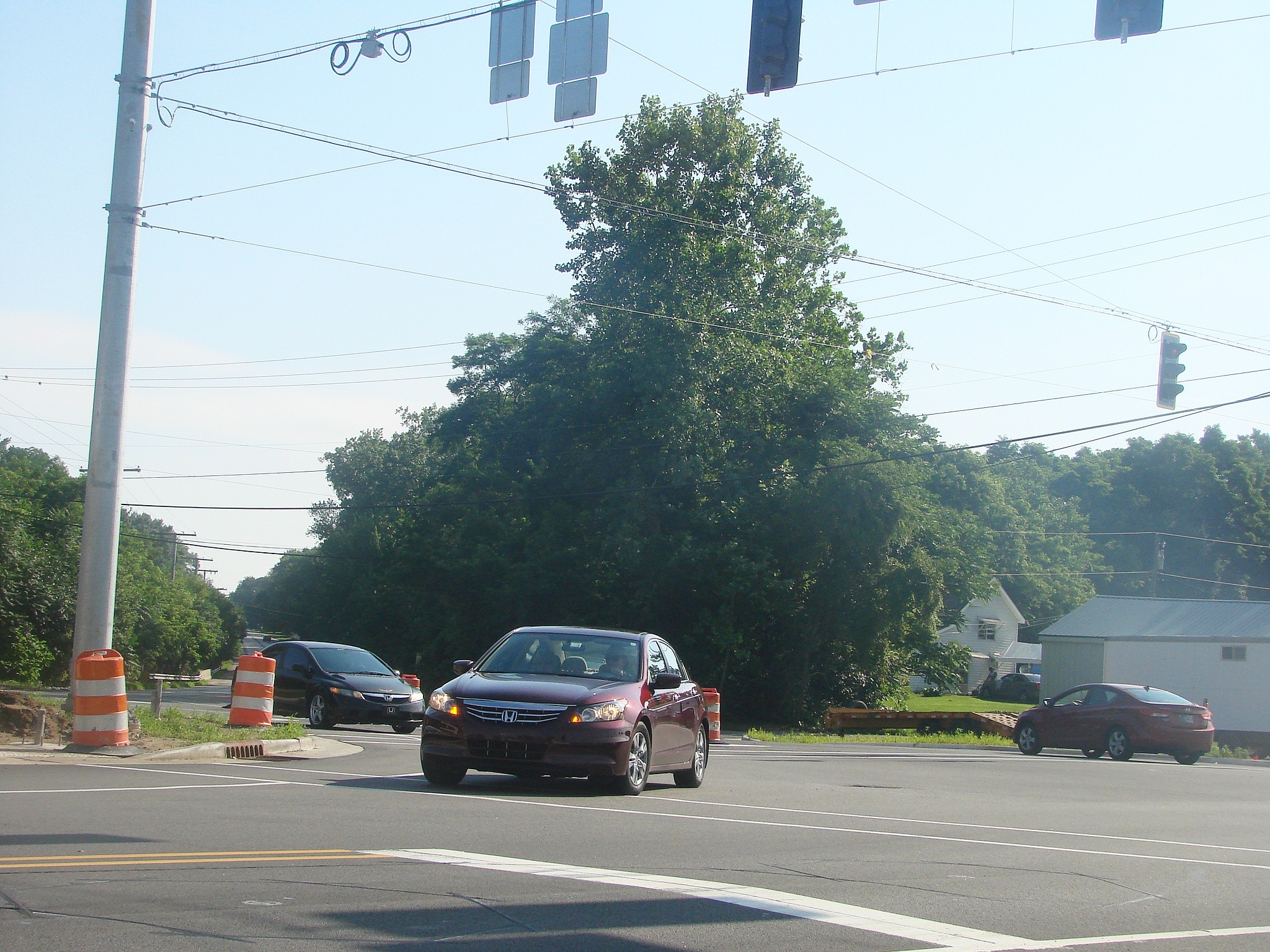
top-left (419, 625), bottom-right (711, 796)
top-left (1012, 683), bottom-right (1216, 765)
top-left (995, 673), bottom-right (1040, 704)
top-left (230, 640), bottom-right (426, 734)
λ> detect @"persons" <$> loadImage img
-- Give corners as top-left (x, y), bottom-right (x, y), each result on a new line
top-left (590, 643), bottom-right (636, 681)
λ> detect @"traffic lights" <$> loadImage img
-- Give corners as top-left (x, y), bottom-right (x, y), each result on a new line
top-left (1156, 331), bottom-right (1187, 410)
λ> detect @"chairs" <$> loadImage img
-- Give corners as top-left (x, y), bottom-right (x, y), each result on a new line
top-left (562, 656), bottom-right (588, 676)
